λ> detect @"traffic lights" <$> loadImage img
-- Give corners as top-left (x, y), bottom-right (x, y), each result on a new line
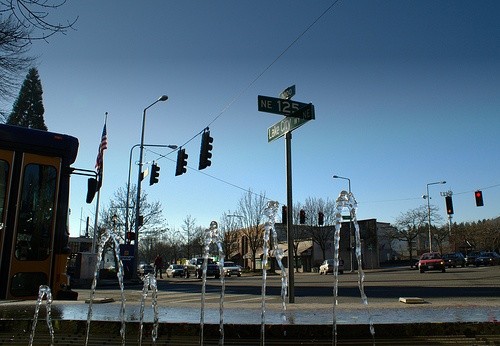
top-left (124, 232), bottom-right (135, 240)
top-left (138, 215), bottom-right (145, 228)
top-left (300, 210), bottom-right (306, 223)
top-left (475, 191), bottom-right (484, 207)
top-left (198, 131), bottom-right (214, 171)
top-left (318, 212), bottom-right (324, 225)
top-left (282, 206), bottom-right (289, 224)
top-left (445, 196), bottom-right (454, 215)
top-left (149, 163), bottom-right (160, 186)
top-left (174, 148), bottom-right (188, 177)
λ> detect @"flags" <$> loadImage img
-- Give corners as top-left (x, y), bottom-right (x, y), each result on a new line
top-left (94, 111), bottom-right (107, 175)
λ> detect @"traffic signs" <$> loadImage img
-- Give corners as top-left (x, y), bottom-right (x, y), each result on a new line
top-left (257, 85), bottom-right (315, 143)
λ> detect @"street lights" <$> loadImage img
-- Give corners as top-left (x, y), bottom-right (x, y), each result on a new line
top-left (333, 175), bottom-right (354, 272)
top-left (423, 182), bottom-right (447, 254)
top-left (124, 95), bottom-right (170, 294)
top-left (228, 214), bottom-right (246, 270)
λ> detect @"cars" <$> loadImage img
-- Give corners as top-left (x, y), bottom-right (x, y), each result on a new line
top-left (318, 259), bottom-right (344, 275)
top-left (136, 262), bottom-right (155, 275)
top-left (412, 252), bottom-right (499, 274)
top-left (166, 257), bottom-right (243, 280)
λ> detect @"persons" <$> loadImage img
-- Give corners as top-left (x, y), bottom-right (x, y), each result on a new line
top-left (153, 254), bottom-right (163, 279)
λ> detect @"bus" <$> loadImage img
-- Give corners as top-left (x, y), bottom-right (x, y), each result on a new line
top-left (0, 123), bottom-right (101, 301)
